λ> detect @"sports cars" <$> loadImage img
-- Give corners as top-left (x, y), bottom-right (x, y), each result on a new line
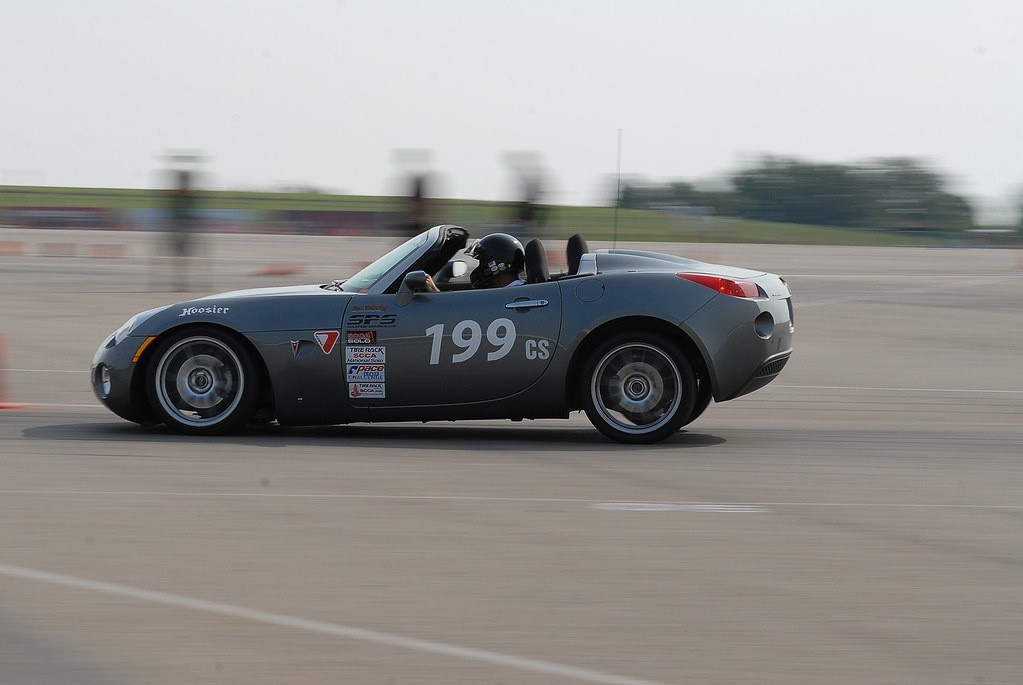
top-left (89, 223), bottom-right (797, 446)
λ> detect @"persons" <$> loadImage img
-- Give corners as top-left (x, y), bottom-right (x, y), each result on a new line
top-left (425, 232), bottom-right (525, 292)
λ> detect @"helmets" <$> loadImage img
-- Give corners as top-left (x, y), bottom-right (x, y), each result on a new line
top-left (470, 233), bottom-right (525, 289)
